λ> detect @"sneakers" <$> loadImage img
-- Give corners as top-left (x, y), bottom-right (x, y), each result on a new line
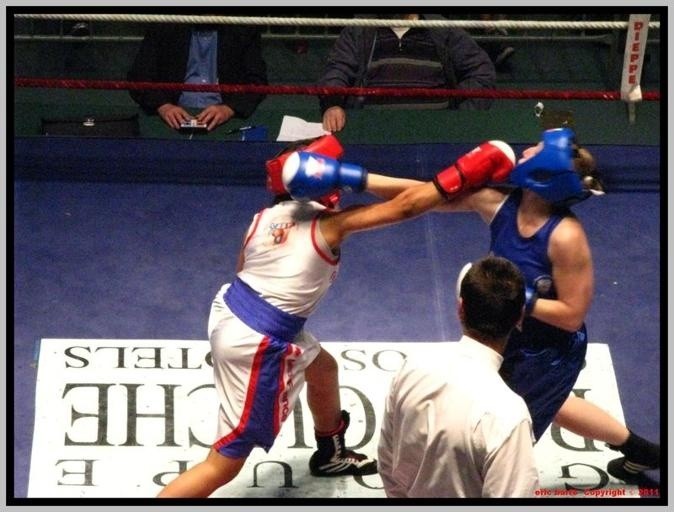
top-left (488, 27), bottom-right (515, 65)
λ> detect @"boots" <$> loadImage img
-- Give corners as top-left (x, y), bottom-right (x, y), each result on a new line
top-left (607, 429), bottom-right (661, 480)
top-left (309, 413), bottom-right (379, 477)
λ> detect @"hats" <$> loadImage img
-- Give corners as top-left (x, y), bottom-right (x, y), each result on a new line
top-left (264, 134), bottom-right (345, 209)
top-left (506, 126), bottom-right (593, 210)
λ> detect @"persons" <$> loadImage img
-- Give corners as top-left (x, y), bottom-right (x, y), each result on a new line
top-left (161, 125), bottom-right (661, 497)
top-left (124, 7), bottom-right (516, 130)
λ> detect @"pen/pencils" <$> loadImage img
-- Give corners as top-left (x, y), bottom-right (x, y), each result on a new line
top-left (227, 125), bottom-right (256, 135)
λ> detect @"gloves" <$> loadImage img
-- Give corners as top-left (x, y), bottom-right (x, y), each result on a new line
top-left (281, 150), bottom-right (366, 202)
top-left (432, 138), bottom-right (516, 202)
